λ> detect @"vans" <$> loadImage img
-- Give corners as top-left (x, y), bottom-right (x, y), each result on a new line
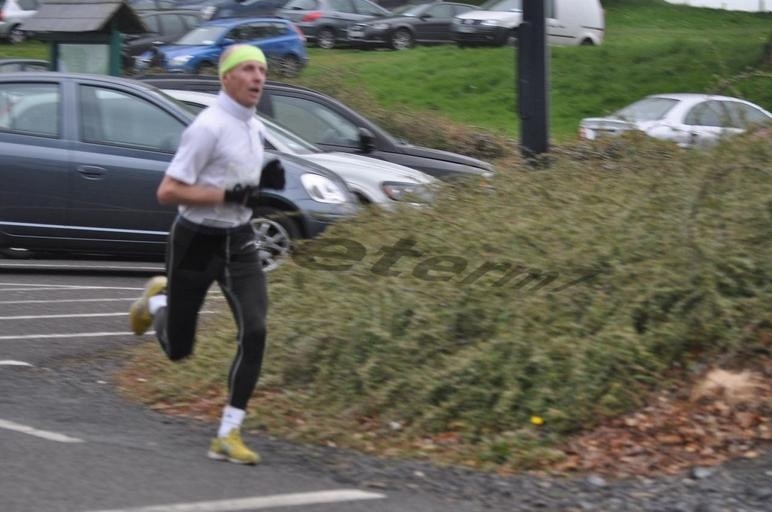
top-left (448, 1), bottom-right (608, 55)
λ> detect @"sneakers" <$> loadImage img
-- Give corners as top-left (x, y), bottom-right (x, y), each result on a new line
top-left (127, 274), bottom-right (169, 337)
top-left (206, 427), bottom-right (262, 467)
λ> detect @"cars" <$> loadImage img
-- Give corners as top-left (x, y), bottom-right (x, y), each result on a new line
top-left (118, 9), bottom-right (203, 71)
top-left (0, 2), bottom-right (42, 45)
top-left (576, 91), bottom-right (771, 153)
top-left (0, 71), bottom-right (361, 275)
top-left (1, 58), bottom-right (57, 92)
top-left (270, 1), bottom-right (394, 51)
top-left (345, 2), bottom-right (478, 50)
top-left (133, 73), bottom-right (497, 190)
top-left (126, 0), bottom-right (293, 26)
top-left (1, 87), bottom-right (456, 216)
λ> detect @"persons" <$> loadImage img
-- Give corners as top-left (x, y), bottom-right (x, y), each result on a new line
top-left (131, 44), bottom-right (285, 465)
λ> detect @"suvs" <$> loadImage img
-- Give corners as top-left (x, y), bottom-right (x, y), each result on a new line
top-left (132, 18), bottom-right (310, 81)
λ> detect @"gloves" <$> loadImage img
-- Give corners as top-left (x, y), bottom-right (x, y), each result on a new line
top-left (257, 156), bottom-right (289, 192)
top-left (222, 181), bottom-right (264, 211)
